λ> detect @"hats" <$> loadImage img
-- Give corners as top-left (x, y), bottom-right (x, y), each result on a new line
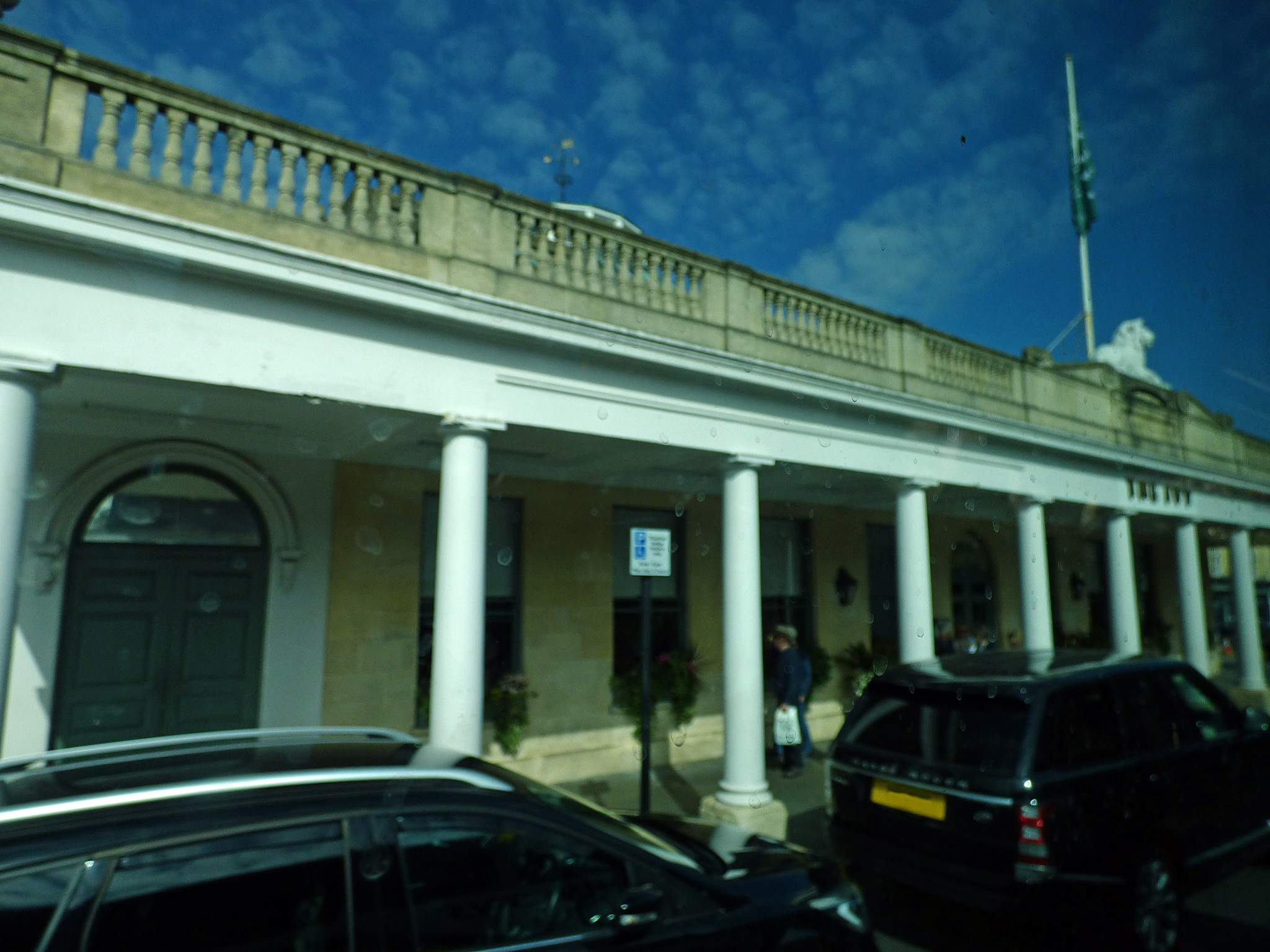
top-left (777, 624), bottom-right (796, 639)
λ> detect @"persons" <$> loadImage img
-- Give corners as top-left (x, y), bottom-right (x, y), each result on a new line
top-left (940, 615), bottom-right (1025, 658)
top-left (768, 625), bottom-right (814, 776)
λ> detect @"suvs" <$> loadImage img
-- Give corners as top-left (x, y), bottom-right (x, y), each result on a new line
top-left (0, 724), bottom-right (873, 952)
top-left (829, 646), bottom-right (1270, 952)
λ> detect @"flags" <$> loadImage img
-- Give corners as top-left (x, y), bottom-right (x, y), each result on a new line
top-left (1067, 99), bottom-right (1096, 235)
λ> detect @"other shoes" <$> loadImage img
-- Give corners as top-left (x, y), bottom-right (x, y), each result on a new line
top-left (783, 767), bottom-right (803, 778)
top-left (809, 749), bottom-right (823, 758)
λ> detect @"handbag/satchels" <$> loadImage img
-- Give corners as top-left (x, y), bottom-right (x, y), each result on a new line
top-left (773, 705), bottom-right (803, 745)
